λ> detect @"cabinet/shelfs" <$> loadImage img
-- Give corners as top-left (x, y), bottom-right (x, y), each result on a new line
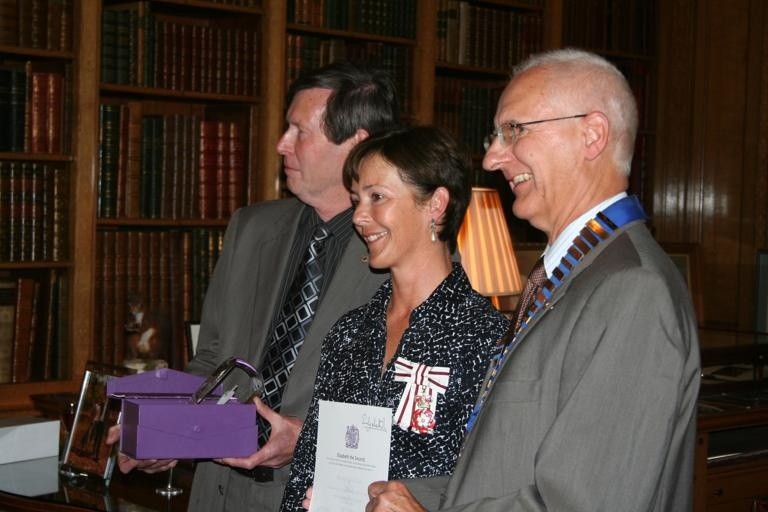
top-left (0, 0), bottom-right (660, 419)
top-left (693, 381), bottom-right (768, 512)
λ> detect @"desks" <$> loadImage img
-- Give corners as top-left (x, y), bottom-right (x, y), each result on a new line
top-left (0, 472), bottom-right (189, 512)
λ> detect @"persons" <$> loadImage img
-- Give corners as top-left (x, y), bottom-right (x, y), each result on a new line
top-left (89, 394), bottom-right (112, 464)
top-left (279, 121), bottom-right (514, 510)
top-left (103, 59), bottom-right (406, 511)
top-left (365, 48), bottom-right (703, 510)
top-left (78, 395), bottom-right (104, 457)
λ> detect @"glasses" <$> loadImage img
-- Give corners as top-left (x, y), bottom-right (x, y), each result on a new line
top-left (482, 112), bottom-right (586, 152)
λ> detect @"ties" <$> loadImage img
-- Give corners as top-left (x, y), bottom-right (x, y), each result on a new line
top-left (503, 256), bottom-right (549, 345)
top-left (257, 223), bottom-right (331, 450)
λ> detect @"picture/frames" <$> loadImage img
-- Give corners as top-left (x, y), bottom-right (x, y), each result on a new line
top-left (63, 359), bottom-right (137, 492)
top-left (659, 242), bottom-right (704, 328)
